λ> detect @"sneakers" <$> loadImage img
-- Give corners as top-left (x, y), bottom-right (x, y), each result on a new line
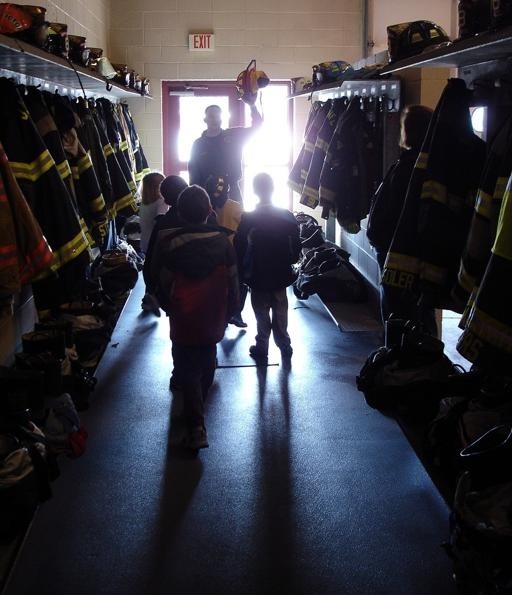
top-left (229, 317), bottom-right (247, 327)
top-left (185, 426), bottom-right (209, 450)
top-left (250, 346), bottom-right (267, 356)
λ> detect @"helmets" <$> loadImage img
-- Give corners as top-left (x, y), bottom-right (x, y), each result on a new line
top-left (313, 61), bottom-right (353, 86)
top-left (386, 20), bottom-right (450, 63)
top-left (2, 3), bottom-right (149, 96)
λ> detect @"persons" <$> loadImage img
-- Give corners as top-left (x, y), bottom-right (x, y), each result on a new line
top-left (135, 168), bottom-right (249, 457)
top-left (186, 89), bottom-right (263, 208)
top-left (364, 103), bottom-right (440, 347)
top-left (234, 171), bottom-right (302, 358)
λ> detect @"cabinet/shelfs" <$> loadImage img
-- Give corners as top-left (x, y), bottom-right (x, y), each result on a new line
top-left (285, 77), bottom-right (402, 335)
top-left (0, 38), bottom-right (156, 595)
top-left (379, 30), bottom-right (512, 594)
